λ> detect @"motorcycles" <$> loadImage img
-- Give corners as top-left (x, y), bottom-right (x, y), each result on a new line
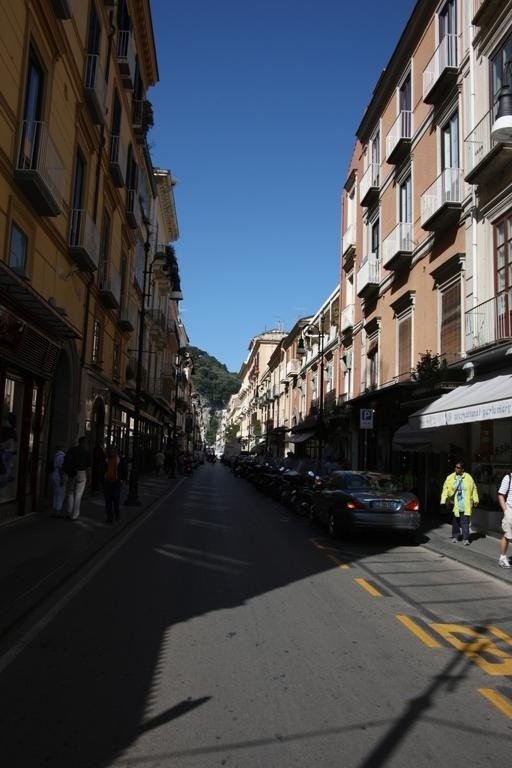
top-left (235, 458), bottom-right (351, 517)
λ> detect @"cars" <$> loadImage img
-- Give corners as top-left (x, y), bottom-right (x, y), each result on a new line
top-left (309, 470), bottom-right (422, 540)
top-left (239, 451), bottom-right (251, 461)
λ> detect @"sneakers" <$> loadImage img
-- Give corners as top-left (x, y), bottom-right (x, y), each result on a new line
top-left (451, 536), bottom-right (471, 546)
top-left (498, 553), bottom-right (511, 569)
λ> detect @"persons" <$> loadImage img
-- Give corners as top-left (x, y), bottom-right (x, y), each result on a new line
top-left (99, 444), bottom-right (127, 522)
top-left (1, 443), bottom-right (16, 482)
top-left (150, 449), bottom-right (194, 479)
top-left (283, 451), bottom-right (354, 482)
top-left (62, 436), bottom-right (92, 520)
top-left (92, 439), bottom-right (129, 494)
top-left (439, 459), bottom-right (480, 546)
top-left (48, 441), bottom-right (66, 519)
top-left (494, 471), bottom-right (512, 571)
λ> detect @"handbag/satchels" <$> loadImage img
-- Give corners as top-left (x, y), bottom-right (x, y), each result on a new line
top-left (444, 495), bottom-right (454, 513)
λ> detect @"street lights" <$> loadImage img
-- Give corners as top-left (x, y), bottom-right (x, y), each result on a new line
top-left (172, 352), bottom-right (204, 475)
top-left (123, 229), bottom-right (183, 506)
top-left (296, 317), bottom-right (325, 476)
